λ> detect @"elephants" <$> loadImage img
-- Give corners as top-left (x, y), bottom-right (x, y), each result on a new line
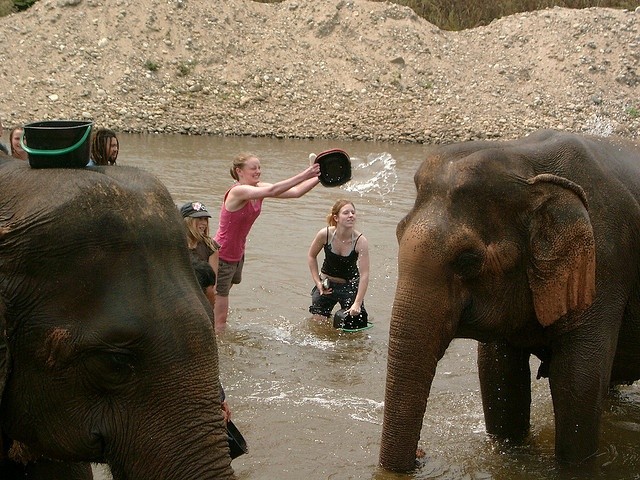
top-left (0, 150), bottom-right (238, 479)
top-left (379, 127), bottom-right (640, 475)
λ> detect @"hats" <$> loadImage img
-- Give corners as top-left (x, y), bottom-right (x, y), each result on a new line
top-left (180, 202), bottom-right (211, 218)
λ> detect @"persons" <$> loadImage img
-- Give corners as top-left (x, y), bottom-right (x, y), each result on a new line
top-left (309, 200), bottom-right (369, 326)
top-left (192, 261), bottom-right (231, 422)
top-left (212, 154), bottom-right (322, 335)
top-left (86, 129), bottom-right (119, 169)
top-left (180, 202), bottom-right (219, 297)
top-left (10, 126), bottom-right (29, 162)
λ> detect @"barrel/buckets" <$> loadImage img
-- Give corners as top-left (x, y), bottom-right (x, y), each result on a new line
top-left (20, 119), bottom-right (93, 168)
